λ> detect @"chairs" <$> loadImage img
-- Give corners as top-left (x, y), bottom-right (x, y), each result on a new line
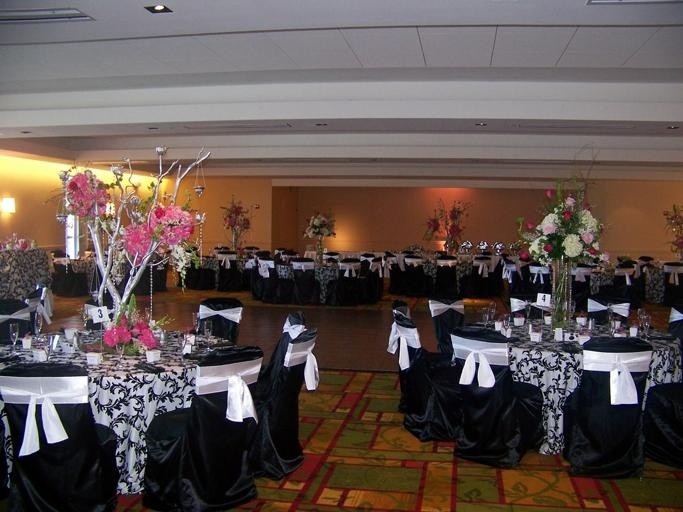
top-left (387, 291), bottom-right (683, 481)
top-left (1, 287), bottom-right (321, 511)
top-left (53, 239), bottom-right (680, 303)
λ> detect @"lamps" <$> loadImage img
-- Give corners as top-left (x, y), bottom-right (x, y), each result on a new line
top-left (0, 195), bottom-right (16, 220)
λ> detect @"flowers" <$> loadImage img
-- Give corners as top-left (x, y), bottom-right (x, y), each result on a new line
top-left (213, 190), bottom-right (253, 232)
top-left (422, 195), bottom-right (475, 253)
top-left (657, 201), bottom-right (683, 262)
top-left (46, 136), bottom-right (219, 361)
top-left (505, 135), bottom-right (603, 269)
top-left (298, 203), bottom-right (337, 241)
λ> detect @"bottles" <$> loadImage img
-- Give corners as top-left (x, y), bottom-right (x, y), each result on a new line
top-left (1, 232), bottom-right (38, 250)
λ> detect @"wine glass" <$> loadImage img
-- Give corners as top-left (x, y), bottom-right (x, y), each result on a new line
top-left (9, 312), bottom-right (214, 364)
top-left (83, 247), bottom-right (124, 261)
top-left (480, 300), bottom-right (652, 345)
